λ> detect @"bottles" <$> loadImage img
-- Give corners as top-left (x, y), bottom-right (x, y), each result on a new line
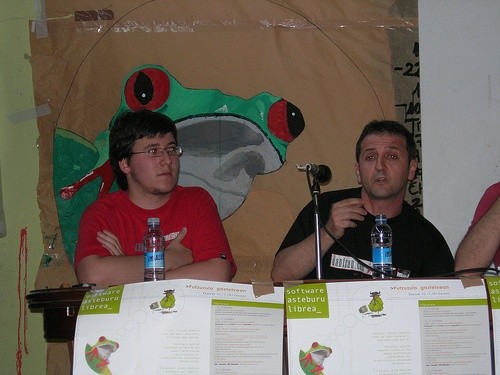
top-left (370, 215), bottom-right (393, 279)
top-left (143, 217), bottom-right (165, 280)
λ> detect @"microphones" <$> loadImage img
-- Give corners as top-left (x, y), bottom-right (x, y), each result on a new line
top-left (306, 164), bottom-right (332, 183)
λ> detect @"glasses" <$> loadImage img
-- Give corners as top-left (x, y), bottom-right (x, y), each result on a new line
top-left (130, 146), bottom-right (182, 158)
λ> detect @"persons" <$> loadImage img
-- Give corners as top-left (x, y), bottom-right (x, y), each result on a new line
top-left (456, 181), bottom-right (500, 277)
top-left (73, 110), bottom-right (237, 292)
top-left (271, 119), bottom-right (455, 284)
